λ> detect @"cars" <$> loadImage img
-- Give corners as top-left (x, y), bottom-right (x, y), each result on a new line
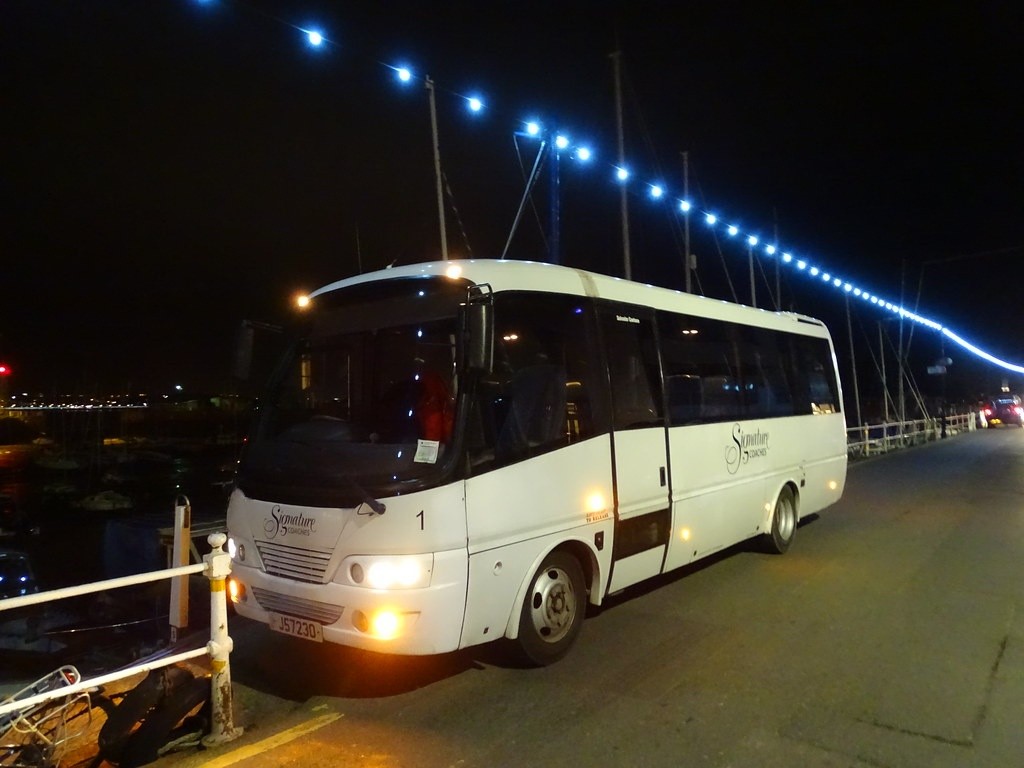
top-left (985, 395), bottom-right (1022, 426)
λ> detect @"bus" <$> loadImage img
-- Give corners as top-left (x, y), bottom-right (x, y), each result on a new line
top-left (226, 256), bottom-right (849, 668)
